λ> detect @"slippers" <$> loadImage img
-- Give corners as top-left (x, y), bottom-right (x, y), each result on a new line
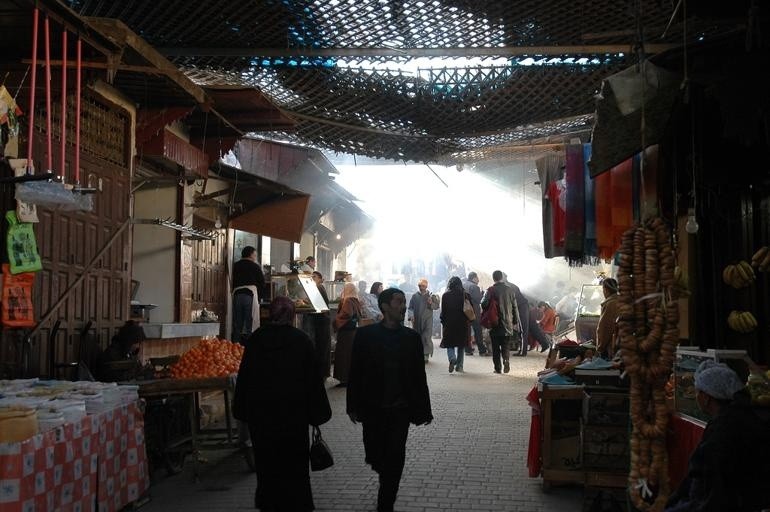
top-left (537, 354), bottom-right (612, 385)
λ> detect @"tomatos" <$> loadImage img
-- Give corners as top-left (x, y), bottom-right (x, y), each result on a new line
top-left (171, 337), bottom-right (245, 378)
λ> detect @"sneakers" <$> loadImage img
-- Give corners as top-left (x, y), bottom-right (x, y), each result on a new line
top-left (514, 353), bottom-right (527, 356)
top-left (493, 359), bottom-right (509, 374)
top-left (541, 345), bottom-right (549, 352)
top-left (466, 352), bottom-right (492, 356)
top-left (449, 359), bottom-right (463, 372)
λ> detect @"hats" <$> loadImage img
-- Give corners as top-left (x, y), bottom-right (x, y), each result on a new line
top-left (694, 360), bottom-right (746, 400)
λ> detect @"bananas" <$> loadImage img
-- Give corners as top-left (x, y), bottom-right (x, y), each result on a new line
top-left (749, 248), bottom-right (769, 273)
top-left (727, 309), bottom-right (759, 332)
top-left (718, 260), bottom-right (755, 289)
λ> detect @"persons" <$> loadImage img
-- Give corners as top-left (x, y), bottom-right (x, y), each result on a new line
top-left (662, 357), bottom-right (770, 512)
top-left (439, 270), bottom-right (580, 373)
top-left (344, 286), bottom-right (435, 511)
top-left (407, 279), bottom-right (440, 362)
top-left (333, 281), bottom-right (384, 387)
top-left (300, 255), bottom-right (316, 275)
top-left (93, 320), bottom-right (146, 383)
top-left (312, 272), bottom-right (329, 306)
top-left (229, 245), bottom-right (266, 343)
top-left (595, 278), bottom-right (620, 361)
top-left (230, 295), bottom-right (333, 511)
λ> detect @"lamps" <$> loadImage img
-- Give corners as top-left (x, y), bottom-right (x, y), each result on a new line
top-left (215, 168), bottom-right (222, 229)
top-left (684, 206), bottom-right (700, 233)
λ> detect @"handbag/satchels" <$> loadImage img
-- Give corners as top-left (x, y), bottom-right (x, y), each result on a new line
top-left (463, 299), bottom-right (477, 321)
top-left (428, 292), bottom-right (440, 309)
top-left (310, 433), bottom-right (333, 471)
top-left (480, 299), bottom-right (499, 327)
top-left (343, 318), bottom-right (357, 332)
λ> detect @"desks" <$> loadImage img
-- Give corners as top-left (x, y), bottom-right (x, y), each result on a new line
top-left (0, 377), bottom-right (151, 512)
top-left (127, 377), bottom-right (240, 482)
top-left (537, 386), bottom-right (585, 494)
